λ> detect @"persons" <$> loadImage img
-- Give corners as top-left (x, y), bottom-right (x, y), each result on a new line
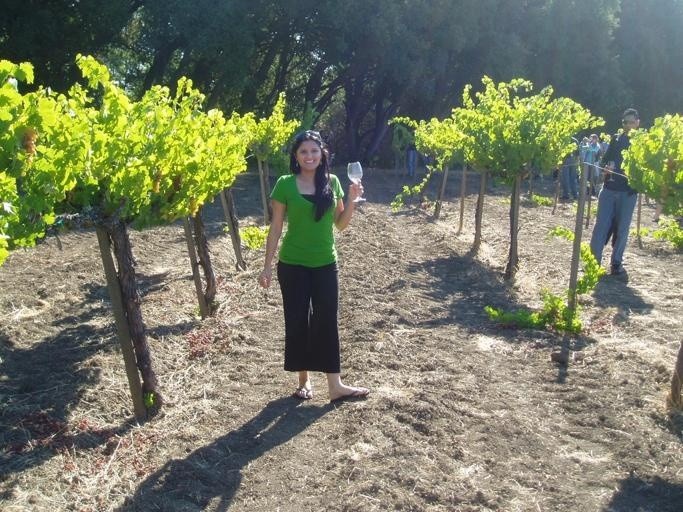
top-left (408, 128), bottom-right (419, 175)
top-left (580, 108), bottom-right (645, 274)
top-left (259, 130), bottom-right (370, 402)
top-left (560, 134), bottom-right (618, 199)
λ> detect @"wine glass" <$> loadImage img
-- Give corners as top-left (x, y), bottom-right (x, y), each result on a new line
top-left (607, 160), bottom-right (617, 183)
top-left (346, 162), bottom-right (368, 203)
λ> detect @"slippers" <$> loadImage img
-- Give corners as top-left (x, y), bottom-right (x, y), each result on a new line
top-left (295, 386), bottom-right (313, 399)
top-left (331, 386), bottom-right (370, 403)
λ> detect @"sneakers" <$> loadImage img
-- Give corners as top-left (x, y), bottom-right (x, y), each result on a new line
top-left (612, 263), bottom-right (624, 274)
